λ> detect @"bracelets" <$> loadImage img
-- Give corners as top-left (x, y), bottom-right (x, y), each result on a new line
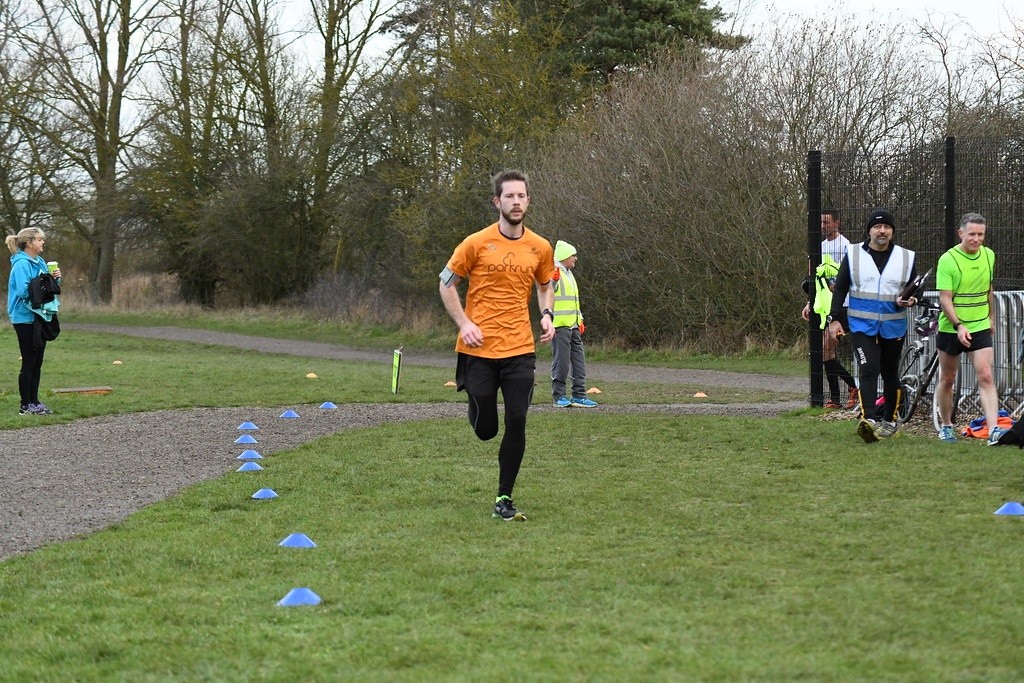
top-left (807, 302), bottom-right (810, 303)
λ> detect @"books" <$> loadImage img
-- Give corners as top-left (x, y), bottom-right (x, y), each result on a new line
top-left (901, 268), bottom-right (933, 299)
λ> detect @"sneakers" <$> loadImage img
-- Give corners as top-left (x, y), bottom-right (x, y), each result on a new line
top-left (875, 420), bottom-right (898, 439)
top-left (986, 427), bottom-right (1010, 446)
top-left (856, 419), bottom-right (878, 443)
top-left (492, 495), bottom-right (526, 521)
top-left (826, 400), bottom-right (841, 409)
top-left (19, 403), bottom-right (46, 415)
top-left (36, 402), bottom-right (53, 414)
top-left (570, 397), bottom-right (598, 408)
top-left (845, 387), bottom-right (859, 409)
top-left (939, 424), bottom-right (957, 442)
top-left (552, 397), bottom-right (572, 408)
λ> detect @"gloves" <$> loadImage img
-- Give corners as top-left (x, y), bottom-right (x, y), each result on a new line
top-left (579, 322), bottom-right (584, 335)
top-left (552, 266), bottom-right (560, 281)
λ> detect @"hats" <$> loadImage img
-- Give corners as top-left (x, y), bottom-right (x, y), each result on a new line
top-left (867, 209), bottom-right (895, 234)
top-left (554, 240), bottom-right (577, 262)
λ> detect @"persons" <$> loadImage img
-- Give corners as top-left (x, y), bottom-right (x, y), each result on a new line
top-left (803, 209), bottom-right (859, 410)
top-left (828, 210), bottom-right (922, 441)
top-left (440, 169), bottom-right (556, 523)
top-left (934, 213), bottom-right (1011, 447)
top-left (5, 228), bottom-right (62, 414)
top-left (552, 241), bottom-right (598, 408)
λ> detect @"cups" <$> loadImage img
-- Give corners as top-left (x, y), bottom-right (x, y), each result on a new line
top-left (46, 261), bottom-right (59, 279)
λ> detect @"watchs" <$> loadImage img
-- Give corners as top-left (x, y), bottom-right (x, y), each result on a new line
top-left (541, 309), bottom-right (554, 323)
top-left (953, 323), bottom-right (961, 331)
top-left (827, 316), bottom-right (839, 323)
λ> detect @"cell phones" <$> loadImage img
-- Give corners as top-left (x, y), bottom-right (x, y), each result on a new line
top-left (902, 300), bottom-right (909, 304)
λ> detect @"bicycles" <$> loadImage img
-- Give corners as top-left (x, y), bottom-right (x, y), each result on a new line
top-left (896, 298), bottom-right (962, 432)
